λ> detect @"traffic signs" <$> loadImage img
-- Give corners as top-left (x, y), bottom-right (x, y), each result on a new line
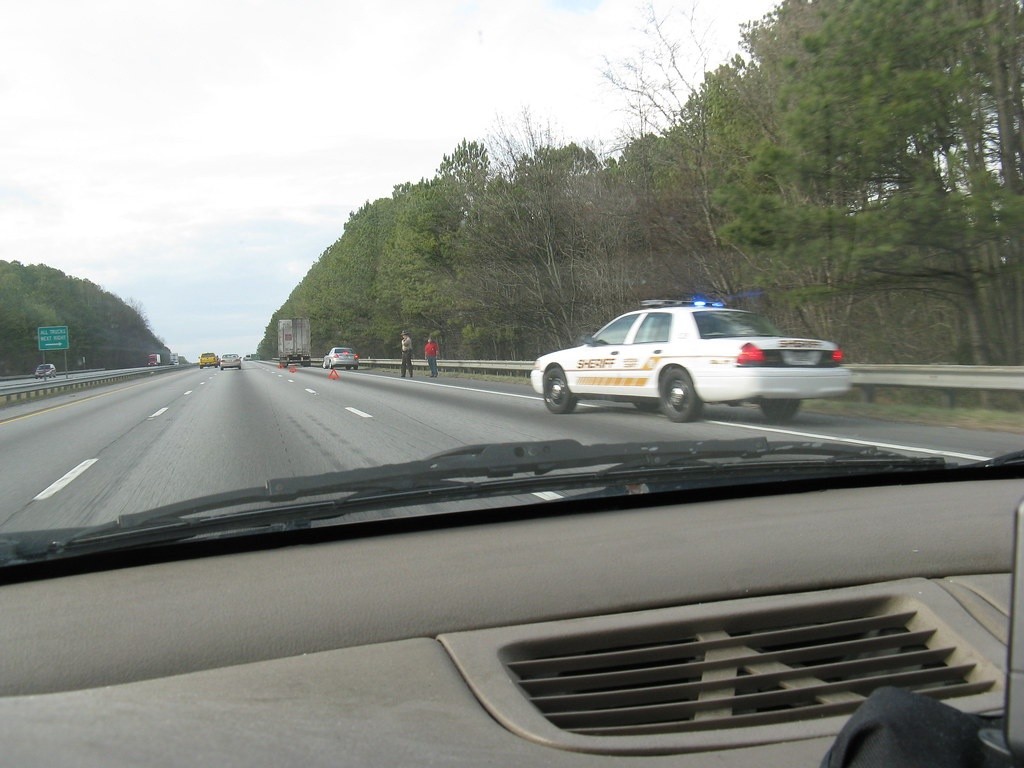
top-left (37, 325), bottom-right (69, 351)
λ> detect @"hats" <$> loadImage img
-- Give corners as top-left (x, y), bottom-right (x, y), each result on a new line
top-left (400, 329), bottom-right (408, 335)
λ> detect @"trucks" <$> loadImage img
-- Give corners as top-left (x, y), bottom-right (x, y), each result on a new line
top-left (146, 353), bottom-right (160, 366)
top-left (277, 316), bottom-right (312, 368)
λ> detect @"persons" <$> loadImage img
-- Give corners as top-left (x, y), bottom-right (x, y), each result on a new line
top-left (401, 330), bottom-right (413, 378)
top-left (425, 337), bottom-right (439, 378)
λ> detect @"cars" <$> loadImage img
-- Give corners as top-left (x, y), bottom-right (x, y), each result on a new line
top-left (35, 364), bottom-right (56, 379)
top-left (198, 352), bottom-right (218, 369)
top-left (530, 299), bottom-right (852, 423)
top-left (322, 347), bottom-right (359, 370)
top-left (169, 361), bottom-right (179, 365)
top-left (220, 353), bottom-right (242, 370)
